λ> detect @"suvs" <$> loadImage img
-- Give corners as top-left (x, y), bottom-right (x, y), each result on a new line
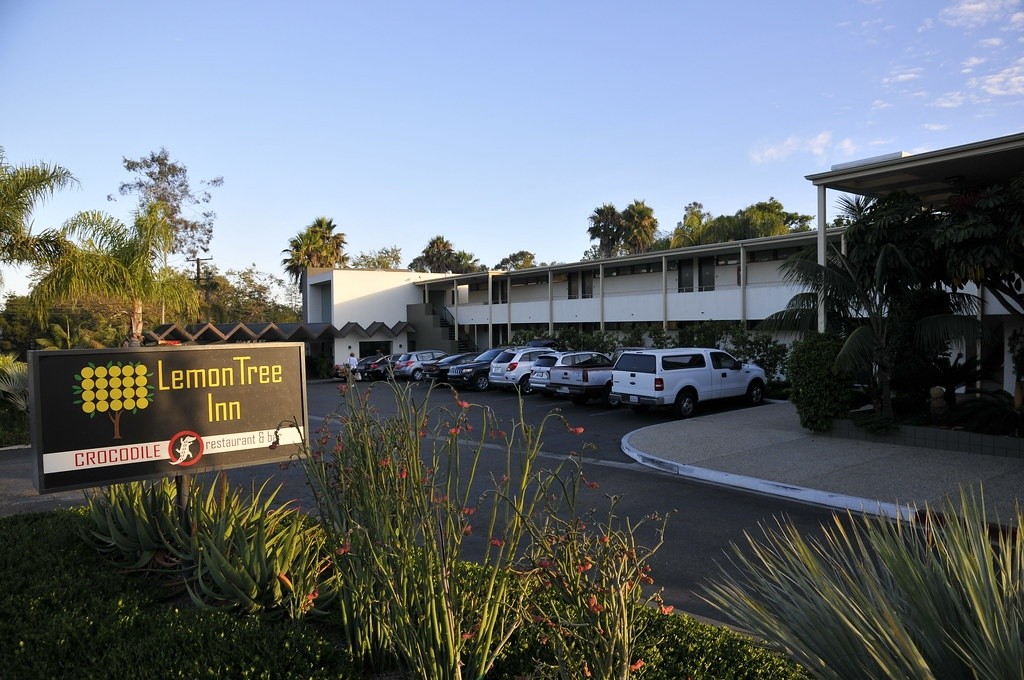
top-left (446, 348), bottom-right (517, 392)
top-left (608, 348), bottom-right (767, 419)
top-left (394, 350), bottom-right (449, 381)
top-left (529, 352), bottom-right (573, 401)
top-left (489, 348), bottom-right (559, 395)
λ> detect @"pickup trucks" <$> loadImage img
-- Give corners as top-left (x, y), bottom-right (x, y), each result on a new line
top-left (545, 348), bottom-right (643, 407)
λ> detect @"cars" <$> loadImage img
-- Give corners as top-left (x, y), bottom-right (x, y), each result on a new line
top-left (334, 356), bottom-right (379, 382)
top-left (422, 351), bottom-right (483, 388)
top-left (365, 354), bottom-right (401, 381)
